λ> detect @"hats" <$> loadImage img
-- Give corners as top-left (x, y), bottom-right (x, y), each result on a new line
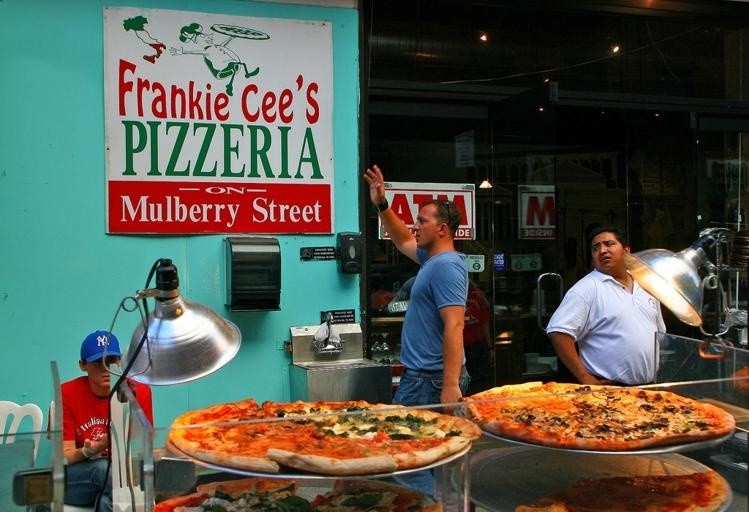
top-left (81, 329), bottom-right (122, 365)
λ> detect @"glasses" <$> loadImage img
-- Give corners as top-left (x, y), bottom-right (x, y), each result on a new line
top-left (441, 199), bottom-right (451, 225)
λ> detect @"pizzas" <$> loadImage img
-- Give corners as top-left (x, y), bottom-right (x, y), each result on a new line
top-left (152, 477), bottom-right (443, 511)
top-left (169, 398), bottom-right (480, 473)
top-left (456, 382), bottom-right (737, 452)
top-left (514, 471), bottom-right (729, 512)
top-left (211, 24), bottom-right (268, 38)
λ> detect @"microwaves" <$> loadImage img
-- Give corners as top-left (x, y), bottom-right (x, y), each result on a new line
top-left (369, 216), bottom-right (396, 270)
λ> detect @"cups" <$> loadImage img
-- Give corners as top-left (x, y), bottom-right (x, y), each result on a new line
top-left (524, 352), bottom-right (541, 373)
top-left (530, 288), bottom-right (546, 316)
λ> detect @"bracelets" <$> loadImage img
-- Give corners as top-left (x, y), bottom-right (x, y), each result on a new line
top-left (372, 199), bottom-right (388, 213)
top-left (81, 446), bottom-right (90, 458)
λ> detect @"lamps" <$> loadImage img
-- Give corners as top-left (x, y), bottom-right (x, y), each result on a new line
top-left (94, 257), bottom-right (243, 512)
top-left (621, 226), bottom-right (749, 345)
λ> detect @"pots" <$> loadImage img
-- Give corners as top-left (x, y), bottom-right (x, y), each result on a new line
top-left (728, 325), bottom-right (748, 345)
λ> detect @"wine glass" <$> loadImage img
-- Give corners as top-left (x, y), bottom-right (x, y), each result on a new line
top-left (381, 333), bottom-right (393, 361)
top-left (371, 332), bottom-right (382, 362)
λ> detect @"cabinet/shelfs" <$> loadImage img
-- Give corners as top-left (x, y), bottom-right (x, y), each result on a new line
top-left (110, 329), bottom-right (748, 512)
top-left (0, 361), bottom-right (64, 512)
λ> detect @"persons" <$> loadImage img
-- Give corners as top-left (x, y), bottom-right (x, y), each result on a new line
top-left (46, 330), bottom-right (156, 512)
top-left (545, 227), bottom-right (668, 385)
top-left (361, 162), bottom-right (472, 493)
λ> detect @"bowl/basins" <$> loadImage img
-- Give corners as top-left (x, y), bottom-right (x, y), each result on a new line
top-left (726, 430), bottom-right (749, 464)
top-left (724, 228), bottom-right (748, 270)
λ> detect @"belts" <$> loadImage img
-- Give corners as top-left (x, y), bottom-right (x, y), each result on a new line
top-left (404, 367), bottom-right (464, 377)
